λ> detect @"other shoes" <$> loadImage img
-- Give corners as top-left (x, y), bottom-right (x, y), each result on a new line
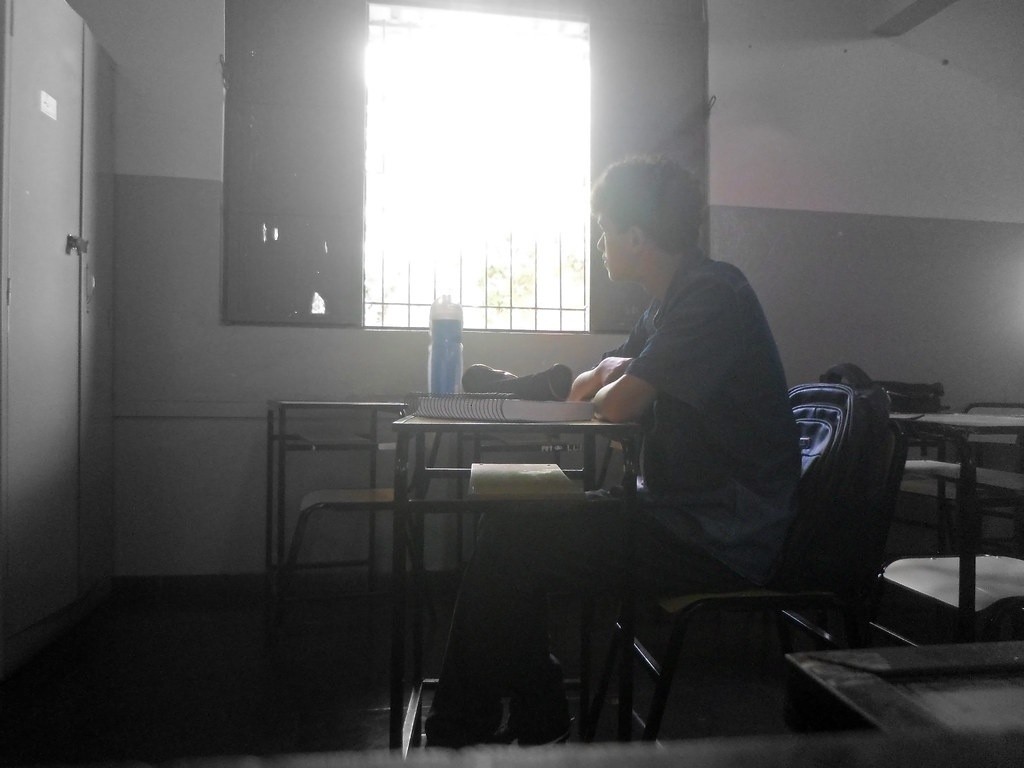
top-left (493, 712), bottom-right (576, 748)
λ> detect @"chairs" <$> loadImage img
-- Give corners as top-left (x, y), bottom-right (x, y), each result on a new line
top-left (582, 385), bottom-right (905, 745)
top-left (905, 398), bottom-right (1024, 556)
top-left (902, 402), bottom-right (1024, 556)
top-left (867, 553), bottom-right (1024, 648)
top-left (283, 430), bottom-right (443, 601)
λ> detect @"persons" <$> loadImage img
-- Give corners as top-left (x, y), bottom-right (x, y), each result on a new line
top-left (425, 160), bottom-right (802, 750)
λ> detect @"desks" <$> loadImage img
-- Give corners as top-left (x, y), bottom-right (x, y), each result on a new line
top-left (387, 412), bottom-right (647, 763)
top-left (888, 413), bottom-right (1024, 645)
top-left (265, 400), bottom-right (410, 601)
top-left (904, 454), bottom-right (1024, 556)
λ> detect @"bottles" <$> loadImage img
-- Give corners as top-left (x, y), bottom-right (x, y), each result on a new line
top-left (427, 293), bottom-right (463, 394)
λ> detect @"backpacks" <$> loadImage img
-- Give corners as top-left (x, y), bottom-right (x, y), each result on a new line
top-left (788, 364), bottom-right (891, 591)
